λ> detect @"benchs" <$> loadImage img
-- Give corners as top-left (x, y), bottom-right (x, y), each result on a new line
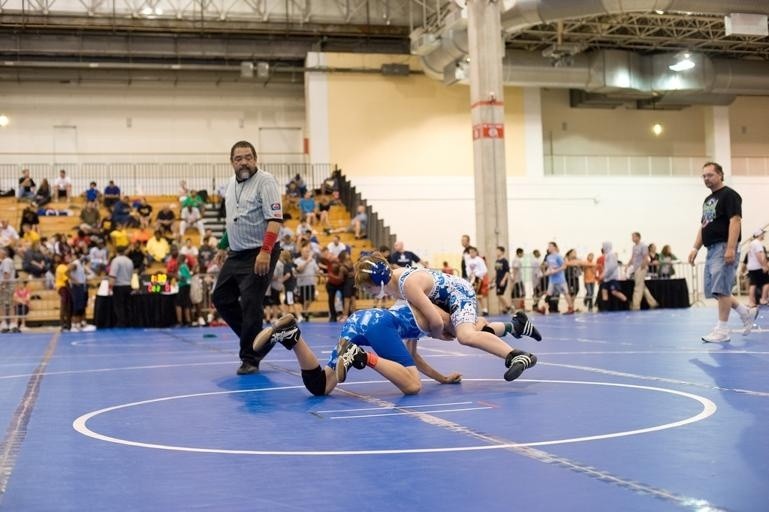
top-left (0, 194), bottom-right (226, 327)
top-left (282, 194), bottom-right (375, 264)
top-left (264, 273), bottom-right (392, 322)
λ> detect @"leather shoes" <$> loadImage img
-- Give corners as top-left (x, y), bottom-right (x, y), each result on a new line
top-left (236, 358), bottom-right (260, 375)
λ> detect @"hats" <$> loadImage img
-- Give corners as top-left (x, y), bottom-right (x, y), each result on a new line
top-left (752, 227), bottom-right (768, 237)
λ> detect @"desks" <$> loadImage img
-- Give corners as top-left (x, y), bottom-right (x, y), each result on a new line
top-left (596, 279), bottom-right (689, 312)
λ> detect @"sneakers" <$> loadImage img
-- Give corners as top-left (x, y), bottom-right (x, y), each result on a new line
top-left (335, 338), bottom-right (367, 382)
top-left (739, 307), bottom-right (759, 335)
top-left (253, 313), bottom-right (301, 353)
top-left (700, 330), bottom-right (731, 343)
top-left (512, 310), bottom-right (542, 342)
top-left (179, 314), bottom-right (314, 325)
top-left (504, 350), bottom-right (537, 381)
top-left (61, 324), bottom-right (98, 333)
top-left (478, 306), bottom-right (600, 316)
